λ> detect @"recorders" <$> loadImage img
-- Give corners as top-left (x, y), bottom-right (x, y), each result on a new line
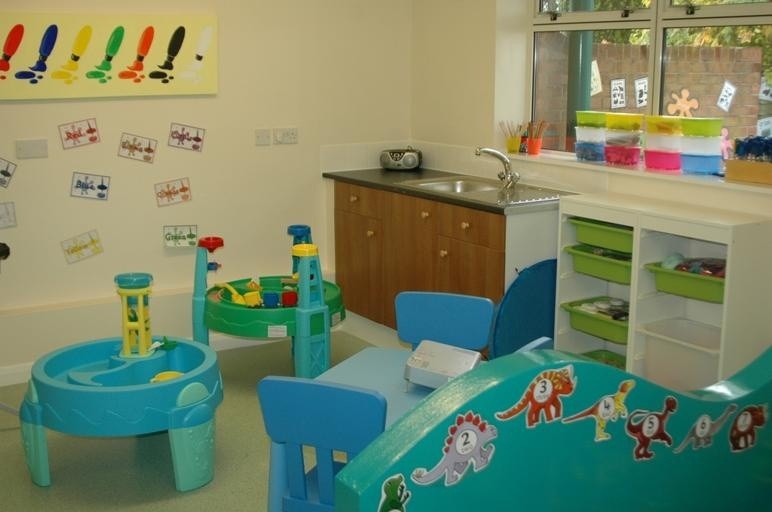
top-left (379, 148), bottom-right (422, 170)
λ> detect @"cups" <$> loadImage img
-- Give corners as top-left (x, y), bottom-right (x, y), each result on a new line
top-left (527, 138), bottom-right (542, 154)
top-left (505, 136), bottom-right (521, 155)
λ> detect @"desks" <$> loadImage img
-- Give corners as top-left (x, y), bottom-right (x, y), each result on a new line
top-left (267, 347), bottom-right (489, 512)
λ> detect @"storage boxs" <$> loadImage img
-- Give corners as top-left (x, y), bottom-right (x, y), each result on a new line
top-left (644, 114), bottom-right (683, 135)
top-left (560, 296), bottom-right (628, 345)
top-left (636, 316), bottom-right (721, 392)
top-left (643, 257), bottom-right (725, 304)
top-left (680, 154), bottom-right (724, 175)
top-left (576, 110), bottom-right (605, 128)
top-left (603, 146), bottom-right (642, 168)
top-left (644, 149), bottom-right (681, 169)
top-left (605, 112), bottom-right (644, 132)
top-left (644, 132), bottom-right (681, 151)
top-left (680, 116), bottom-right (724, 136)
top-left (574, 142), bottom-right (606, 164)
top-left (567, 216), bottom-right (634, 254)
top-left (604, 129), bottom-right (645, 147)
top-left (581, 349), bottom-right (626, 371)
top-left (680, 135), bottom-right (723, 155)
top-left (562, 244), bottom-right (632, 285)
top-left (575, 127), bottom-right (605, 144)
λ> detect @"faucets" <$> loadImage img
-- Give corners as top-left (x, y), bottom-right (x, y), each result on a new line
top-left (474, 145), bottom-right (521, 189)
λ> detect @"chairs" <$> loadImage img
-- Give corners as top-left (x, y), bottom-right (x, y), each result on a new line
top-left (394, 290), bottom-right (495, 352)
top-left (256, 376), bottom-right (387, 511)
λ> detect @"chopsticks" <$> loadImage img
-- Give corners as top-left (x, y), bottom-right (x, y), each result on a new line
top-left (527, 119), bottom-right (548, 138)
top-left (497, 119), bottom-right (527, 136)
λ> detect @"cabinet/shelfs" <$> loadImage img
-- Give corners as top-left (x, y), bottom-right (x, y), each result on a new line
top-left (323, 181), bottom-right (559, 359)
top-left (554, 192), bottom-right (771, 390)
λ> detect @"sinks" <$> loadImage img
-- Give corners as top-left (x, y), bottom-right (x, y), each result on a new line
top-left (396, 174), bottom-right (555, 207)
